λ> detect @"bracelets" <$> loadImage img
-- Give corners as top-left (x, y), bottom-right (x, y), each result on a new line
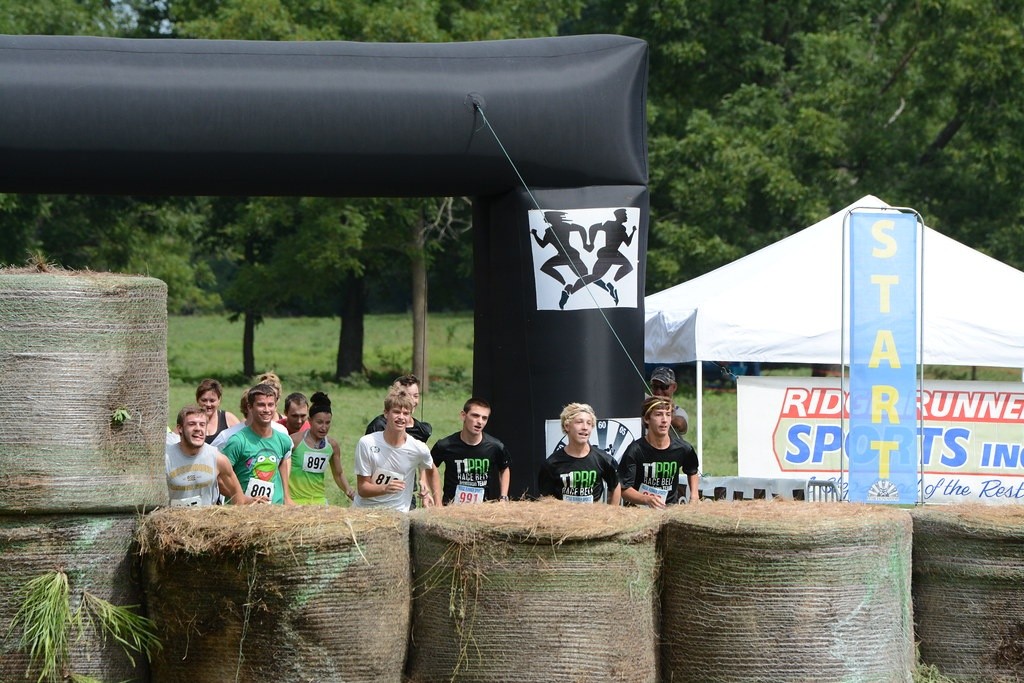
top-left (499, 496), bottom-right (508, 501)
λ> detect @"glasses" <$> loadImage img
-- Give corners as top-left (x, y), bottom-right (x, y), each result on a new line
top-left (652, 384), bottom-right (670, 390)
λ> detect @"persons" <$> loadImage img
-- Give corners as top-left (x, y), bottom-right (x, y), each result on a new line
top-left (164, 373), bottom-right (357, 508)
top-left (537, 402), bottom-right (621, 506)
top-left (645, 366), bottom-right (688, 439)
top-left (421, 398), bottom-right (512, 507)
top-left (164, 405), bottom-right (244, 506)
top-left (618, 397), bottom-right (701, 509)
top-left (351, 392), bottom-right (442, 512)
top-left (365, 375), bottom-right (435, 507)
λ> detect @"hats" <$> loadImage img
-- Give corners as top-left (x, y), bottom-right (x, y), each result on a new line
top-left (650, 366), bottom-right (676, 385)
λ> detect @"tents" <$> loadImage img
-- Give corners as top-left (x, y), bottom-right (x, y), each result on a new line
top-left (644, 195), bottom-right (1024, 476)
